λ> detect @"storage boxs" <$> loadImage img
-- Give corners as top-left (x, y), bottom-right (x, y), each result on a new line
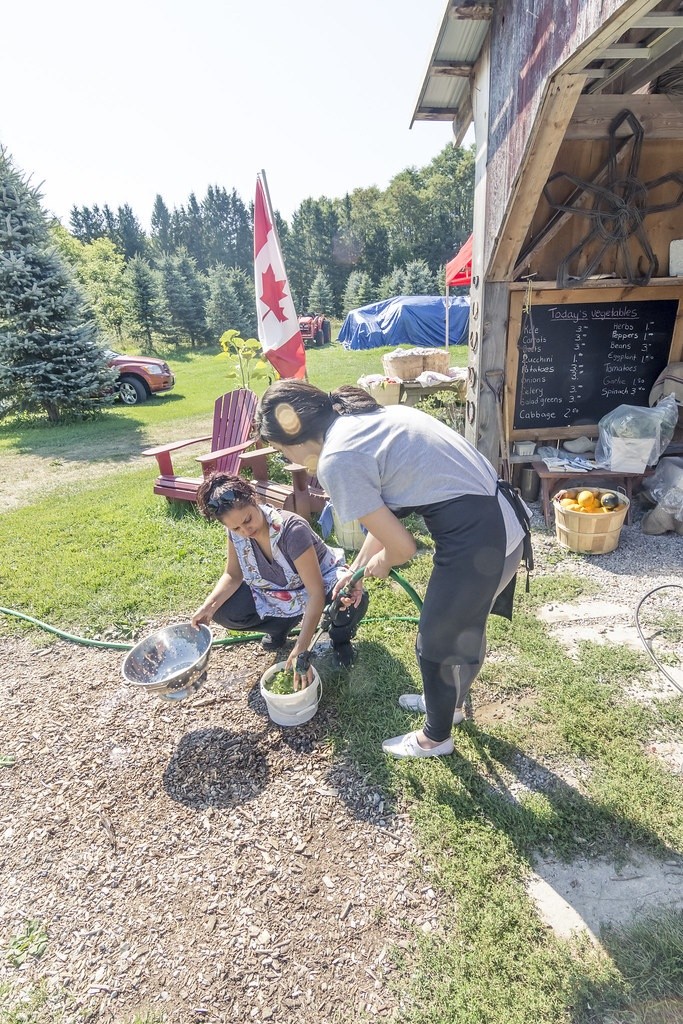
top-left (356, 378), bottom-right (400, 406)
top-left (610, 432), bottom-right (655, 474)
top-left (407, 350), bottom-right (450, 376)
top-left (514, 441), bottom-right (537, 456)
top-left (380, 352), bottom-right (425, 382)
top-left (552, 487), bottom-right (630, 555)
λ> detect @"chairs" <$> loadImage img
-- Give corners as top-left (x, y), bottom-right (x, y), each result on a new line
top-left (141, 388), bottom-right (263, 507)
top-left (237, 447), bottom-right (330, 523)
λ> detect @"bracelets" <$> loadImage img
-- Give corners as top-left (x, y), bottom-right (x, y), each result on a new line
top-left (349, 569), bottom-right (361, 578)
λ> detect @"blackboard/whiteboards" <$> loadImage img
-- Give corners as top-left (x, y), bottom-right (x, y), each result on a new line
top-left (502, 286), bottom-right (682, 441)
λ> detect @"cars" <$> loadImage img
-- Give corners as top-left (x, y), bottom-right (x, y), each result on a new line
top-left (99, 347), bottom-right (176, 405)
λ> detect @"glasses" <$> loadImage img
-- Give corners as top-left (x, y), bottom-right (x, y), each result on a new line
top-left (204, 489), bottom-right (248, 513)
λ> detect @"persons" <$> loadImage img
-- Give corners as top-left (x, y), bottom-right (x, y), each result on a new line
top-left (191, 469), bottom-right (370, 692)
top-left (258, 381), bottom-right (536, 760)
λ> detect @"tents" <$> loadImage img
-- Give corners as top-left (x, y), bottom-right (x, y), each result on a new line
top-left (445, 232), bottom-right (471, 351)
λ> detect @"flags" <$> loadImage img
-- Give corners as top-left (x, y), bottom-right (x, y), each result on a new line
top-left (254, 173), bottom-right (307, 379)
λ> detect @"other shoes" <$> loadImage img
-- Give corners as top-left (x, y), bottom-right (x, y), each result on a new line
top-left (260, 613), bottom-right (303, 650)
top-left (563, 436), bottom-right (598, 453)
top-left (329, 638), bottom-right (353, 667)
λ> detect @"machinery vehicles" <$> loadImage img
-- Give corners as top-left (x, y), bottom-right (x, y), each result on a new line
top-left (295, 294), bottom-right (333, 348)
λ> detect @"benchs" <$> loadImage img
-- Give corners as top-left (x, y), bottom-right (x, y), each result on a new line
top-left (509, 424), bottom-right (601, 492)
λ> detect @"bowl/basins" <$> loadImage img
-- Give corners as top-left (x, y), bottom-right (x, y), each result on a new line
top-left (121, 624), bottom-right (214, 693)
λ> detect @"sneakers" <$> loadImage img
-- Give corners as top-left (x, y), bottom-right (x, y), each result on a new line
top-left (381, 730), bottom-right (455, 760)
top-left (398, 694), bottom-right (463, 725)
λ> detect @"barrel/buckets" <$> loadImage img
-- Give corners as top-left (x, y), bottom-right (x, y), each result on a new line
top-left (327, 501), bottom-right (366, 550)
top-left (259, 660), bottom-right (323, 727)
top-left (552, 488), bottom-right (630, 554)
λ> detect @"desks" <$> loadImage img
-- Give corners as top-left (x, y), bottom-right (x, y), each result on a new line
top-left (531, 459), bottom-right (655, 529)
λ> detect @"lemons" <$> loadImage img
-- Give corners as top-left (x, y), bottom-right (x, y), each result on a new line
top-left (557, 489), bottom-right (626, 514)
